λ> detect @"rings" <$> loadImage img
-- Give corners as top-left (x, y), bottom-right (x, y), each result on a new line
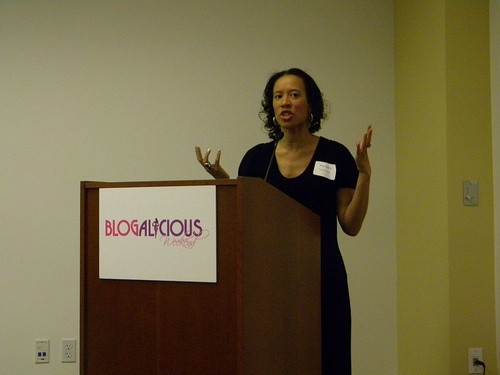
top-left (204, 163), bottom-right (213, 170)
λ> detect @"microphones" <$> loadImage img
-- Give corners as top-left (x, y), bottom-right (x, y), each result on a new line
top-left (262, 132), bottom-right (284, 185)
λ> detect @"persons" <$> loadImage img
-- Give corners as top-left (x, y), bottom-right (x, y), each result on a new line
top-left (195, 68), bottom-right (373, 375)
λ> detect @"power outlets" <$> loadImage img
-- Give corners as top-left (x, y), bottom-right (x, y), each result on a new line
top-left (61, 339), bottom-right (76, 363)
top-left (467, 347), bottom-right (483, 374)
top-left (34, 338), bottom-right (50, 363)
